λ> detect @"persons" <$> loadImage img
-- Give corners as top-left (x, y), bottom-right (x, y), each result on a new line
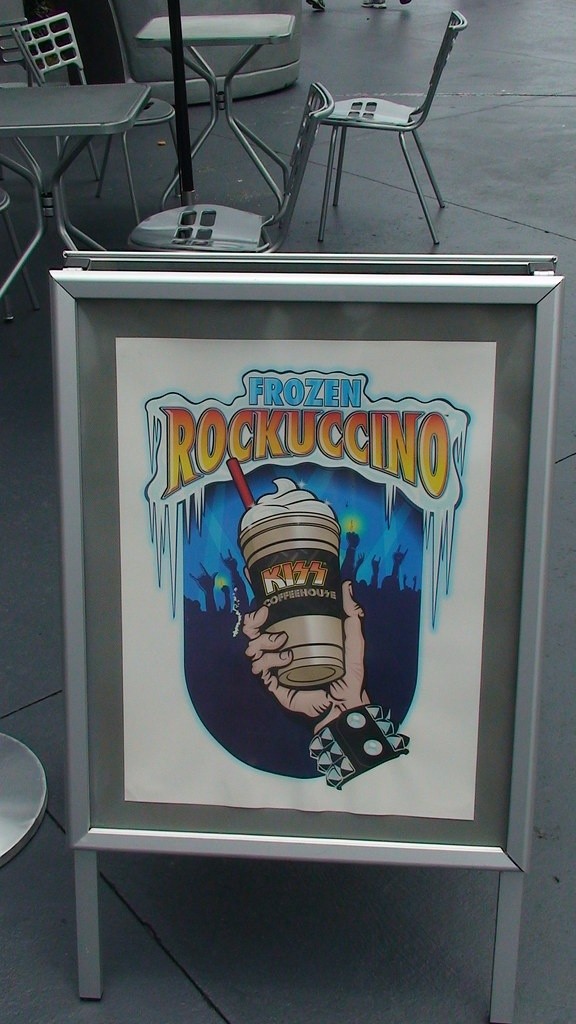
top-left (305, 0), bottom-right (412, 10)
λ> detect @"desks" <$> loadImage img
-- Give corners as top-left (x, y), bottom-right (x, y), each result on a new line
top-left (134, 11), bottom-right (299, 230)
top-left (0, 84), bottom-right (151, 303)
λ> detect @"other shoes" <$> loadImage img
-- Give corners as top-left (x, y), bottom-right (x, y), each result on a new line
top-left (361, 0), bottom-right (386, 9)
top-left (306, 0), bottom-right (326, 10)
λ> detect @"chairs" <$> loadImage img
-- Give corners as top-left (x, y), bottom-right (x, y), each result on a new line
top-left (0, 21), bottom-right (75, 192)
top-left (310, 12), bottom-right (467, 246)
top-left (127, 82), bottom-right (331, 253)
top-left (16, 13), bottom-right (183, 198)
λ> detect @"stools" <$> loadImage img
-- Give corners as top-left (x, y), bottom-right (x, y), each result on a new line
top-left (0, 190), bottom-right (40, 322)
